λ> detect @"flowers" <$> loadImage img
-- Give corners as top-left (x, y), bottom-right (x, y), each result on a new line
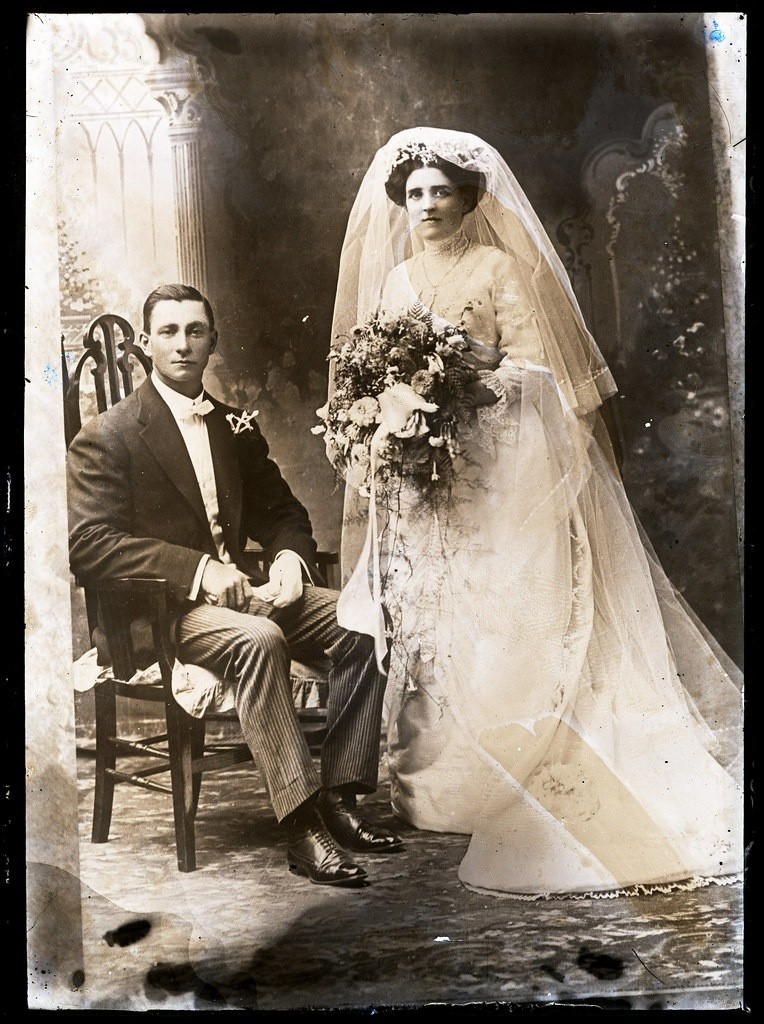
top-left (311, 299), bottom-right (474, 527)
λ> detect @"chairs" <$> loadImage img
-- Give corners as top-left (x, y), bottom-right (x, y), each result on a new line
top-left (59, 314), bottom-right (339, 875)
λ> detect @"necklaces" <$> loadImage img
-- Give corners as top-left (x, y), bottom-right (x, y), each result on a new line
top-left (422, 239), bottom-right (472, 292)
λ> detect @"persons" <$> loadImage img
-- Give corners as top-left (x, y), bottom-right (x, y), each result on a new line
top-left (324, 125), bottom-right (657, 894)
top-left (68, 282), bottom-right (406, 887)
